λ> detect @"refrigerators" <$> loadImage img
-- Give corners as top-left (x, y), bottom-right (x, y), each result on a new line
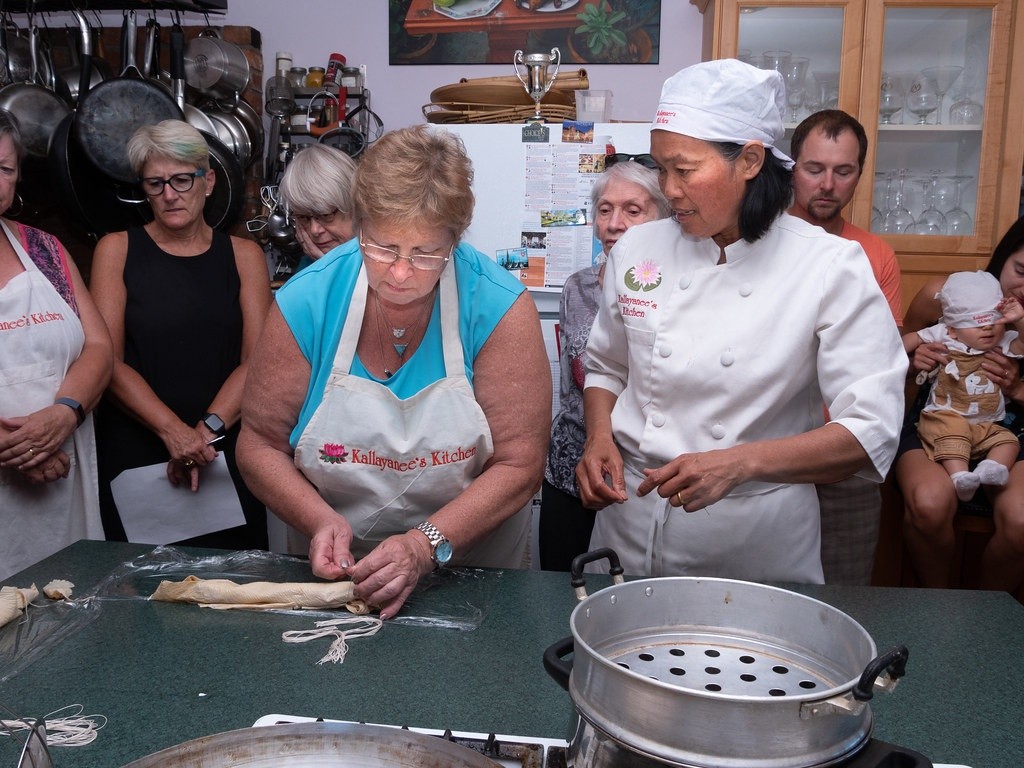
top-left (417, 121), bottom-right (652, 571)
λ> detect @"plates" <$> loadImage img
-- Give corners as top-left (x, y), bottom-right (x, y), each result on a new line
top-left (514, 0), bottom-right (580, 13)
top-left (433, 0), bottom-right (502, 20)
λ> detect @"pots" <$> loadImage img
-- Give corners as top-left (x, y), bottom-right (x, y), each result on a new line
top-left (540, 637), bottom-right (935, 768)
top-left (119, 722), bottom-right (504, 768)
top-left (0, 10), bottom-right (265, 248)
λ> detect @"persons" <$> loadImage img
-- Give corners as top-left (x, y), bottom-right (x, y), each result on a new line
top-left (89, 121), bottom-right (276, 556)
top-left (877, 215), bottom-right (1024, 592)
top-left (775, 107), bottom-right (907, 592)
top-left (902, 268), bottom-right (1023, 502)
top-left (234, 126), bottom-right (553, 624)
top-left (572, 56), bottom-right (911, 588)
top-left (264, 144), bottom-right (362, 555)
top-left (0, 111), bottom-right (115, 582)
top-left (537, 160), bottom-right (673, 570)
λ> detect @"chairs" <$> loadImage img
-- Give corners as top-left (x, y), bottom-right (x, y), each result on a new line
top-left (870, 448), bottom-right (996, 591)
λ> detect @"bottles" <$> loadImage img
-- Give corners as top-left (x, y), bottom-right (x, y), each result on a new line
top-left (274, 52), bottom-right (363, 88)
top-left (291, 99), bottom-right (350, 126)
top-left (278, 142), bottom-right (351, 164)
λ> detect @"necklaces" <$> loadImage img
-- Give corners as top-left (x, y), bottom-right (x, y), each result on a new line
top-left (373, 289), bottom-right (431, 379)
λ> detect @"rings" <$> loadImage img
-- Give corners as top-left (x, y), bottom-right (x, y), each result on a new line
top-left (1001, 368), bottom-right (1008, 378)
top-left (29, 448), bottom-right (36, 457)
top-left (677, 492), bottom-right (688, 505)
top-left (300, 240), bottom-right (305, 245)
top-left (184, 460), bottom-right (194, 467)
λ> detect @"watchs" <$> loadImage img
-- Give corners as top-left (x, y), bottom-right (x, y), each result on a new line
top-left (412, 521), bottom-right (454, 573)
top-left (54, 398), bottom-right (87, 428)
top-left (200, 412), bottom-right (227, 438)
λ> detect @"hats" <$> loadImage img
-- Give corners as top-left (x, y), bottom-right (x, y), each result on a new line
top-left (650, 59), bottom-right (796, 171)
top-left (934, 270), bottom-right (1004, 329)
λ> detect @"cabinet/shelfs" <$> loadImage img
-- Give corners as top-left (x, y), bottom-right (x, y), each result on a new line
top-left (710, 1), bottom-right (1024, 318)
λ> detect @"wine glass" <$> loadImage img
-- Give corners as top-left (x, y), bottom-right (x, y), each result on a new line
top-left (869, 167), bottom-right (976, 236)
top-left (737, 49), bottom-right (840, 123)
top-left (878, 66), bottom-right (984, 126)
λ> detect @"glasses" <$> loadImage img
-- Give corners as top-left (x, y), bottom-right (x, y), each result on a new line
top-left (605, 153), bottom-right (657, 169)
top-left (137, 167), bottom-right (207, 196)
top-left (360, 222), bottom-right (455, 270)
top-left (288, 208), bottom-right (338, 225)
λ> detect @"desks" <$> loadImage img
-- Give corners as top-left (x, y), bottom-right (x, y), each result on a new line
top-left (0, 536), bottom-right (1023, 768)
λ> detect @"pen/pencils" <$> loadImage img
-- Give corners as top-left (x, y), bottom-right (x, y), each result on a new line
top-left (169, 435), bottom-right (226, 461)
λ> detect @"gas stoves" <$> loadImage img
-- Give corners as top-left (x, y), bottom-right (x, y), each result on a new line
top-left (255, 714), bottom-right (571, 768)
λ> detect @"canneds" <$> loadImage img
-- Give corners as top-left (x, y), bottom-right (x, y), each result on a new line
top-left (275, 50), bottom-right (347, 128)
